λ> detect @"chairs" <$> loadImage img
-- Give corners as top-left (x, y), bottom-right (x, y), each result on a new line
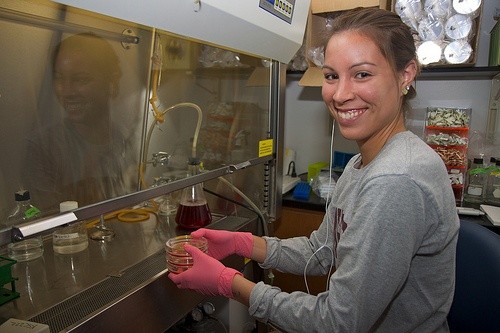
top-left (446, 219), bottom-right (500, 333)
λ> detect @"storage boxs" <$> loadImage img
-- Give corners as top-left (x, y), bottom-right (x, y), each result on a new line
top-left (298, 0), bottom-right (484, 86)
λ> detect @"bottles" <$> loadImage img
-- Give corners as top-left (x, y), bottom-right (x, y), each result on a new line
top-left (486, 157), bottom-right (496, 184)
top-left (52, 201), bottom-right (88, 255)
top-left (484, 159), bottom-right (500, 206)
top-left (8, 190), bottom-right (44, 262)
top-left (175, 159), bottom-right (213, 229)
top-left (463, 158), bottom-right (486, 203)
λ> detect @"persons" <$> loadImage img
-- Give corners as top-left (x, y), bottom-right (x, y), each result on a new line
top-left (166, 6), bottom-right (461, 333)
top-left (21, 32), bottom-right (142, 215)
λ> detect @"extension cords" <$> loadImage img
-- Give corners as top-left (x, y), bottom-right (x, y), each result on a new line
top-left (282, 174), bottom-right (300, 195)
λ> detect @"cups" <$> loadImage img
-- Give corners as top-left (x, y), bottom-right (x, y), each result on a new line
top-left (166, 235), bottom-right (209, 274)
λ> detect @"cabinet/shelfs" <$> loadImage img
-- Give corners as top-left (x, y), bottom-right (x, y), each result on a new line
top-left (423, 106), bottom-right (472, 202)
